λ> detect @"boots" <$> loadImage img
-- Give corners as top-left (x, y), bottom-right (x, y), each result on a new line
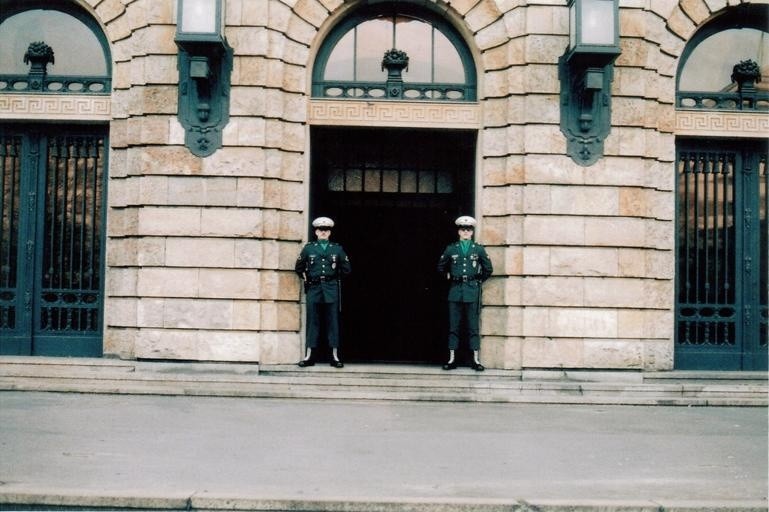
top-left (330, 347), bottom-right (343, 367)
top-left (473, 350), bottom-right (484, 370)
top-left (299, 348), bottom-right (313, 366)
top-left (443, 349), bottom-right (457, 370)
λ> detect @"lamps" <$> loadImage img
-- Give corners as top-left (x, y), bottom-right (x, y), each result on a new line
top-left (175, 0), bottom-right (226, 88)
top-left (560, 3), bottom-right (623, 94)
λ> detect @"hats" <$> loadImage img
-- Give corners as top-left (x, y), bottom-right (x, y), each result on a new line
top-left (455, 216), bottom-right (476, 228)
top-left (312, 217), bottom-right (335, 230)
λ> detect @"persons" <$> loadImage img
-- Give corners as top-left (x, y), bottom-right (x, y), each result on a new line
top-left (295, 217), bottom-right (352, 368)
top-left (438, 216), bottom-right (493, 371)
